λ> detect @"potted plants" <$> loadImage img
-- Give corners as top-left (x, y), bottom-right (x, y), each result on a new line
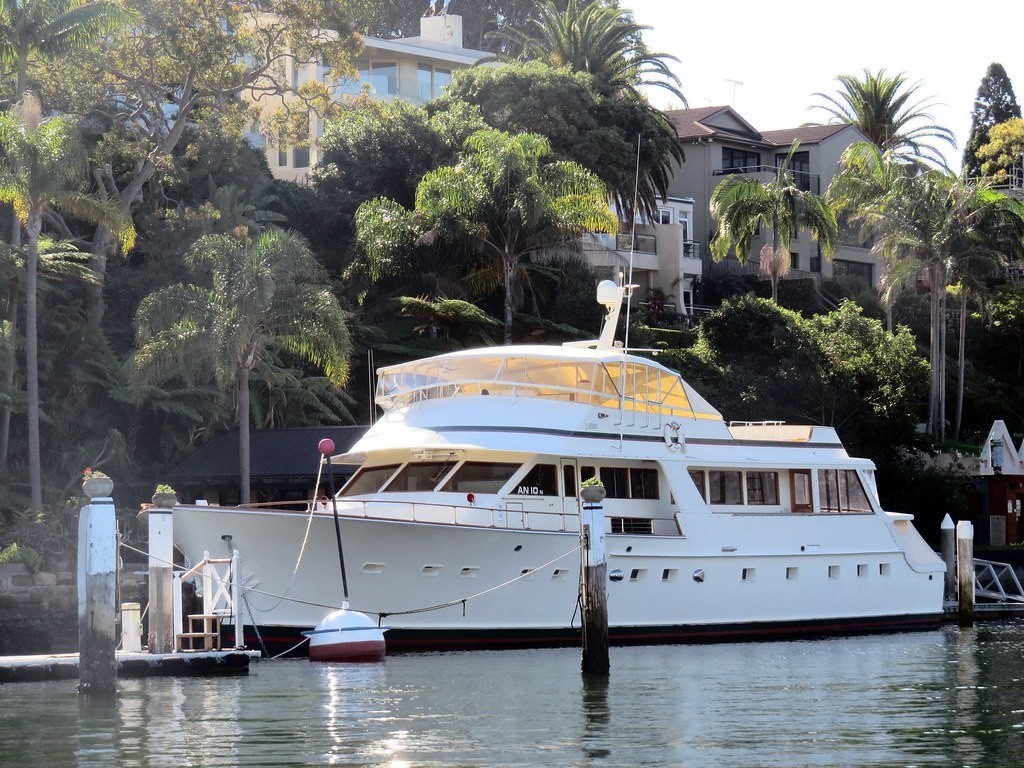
top-left (152, 484), bottom-right (177, 508)
top-left (82, 467), bottom-right (114, 497)
top-left (580, 476), bottom-right (606, 503)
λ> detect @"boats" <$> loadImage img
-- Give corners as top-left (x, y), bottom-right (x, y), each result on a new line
top-left (136, 271), bottom-right (948, 650)
